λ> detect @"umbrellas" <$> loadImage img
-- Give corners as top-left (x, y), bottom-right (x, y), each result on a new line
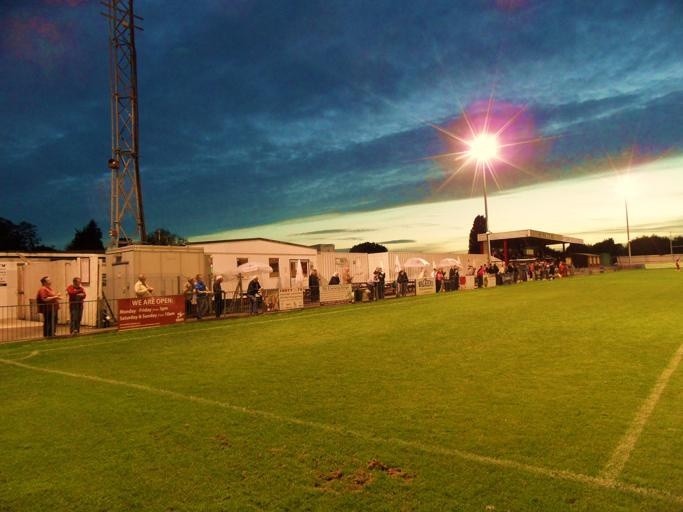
top-left (295, 258), bottom-right (305, 283)
top-left (404, 258), bottom-right (429, 267)
top-left (237, 262), bottom-right (273, 273)
top-left (394, 255), bottom-right (402, 272)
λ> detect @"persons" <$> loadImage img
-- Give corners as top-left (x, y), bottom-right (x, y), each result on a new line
top-left (67, 278), bottom-right (86, 335)
top-left (182, 277), bottom-right (195, 319)
top-left (675, 254), bottom-right (681, 271)
top-left (213, 276), bottom-right (226, 320)
top-left (307, 267), bottom-right (408, 300)
top-left (436, 257), bottom-right (575, 293)
top-left (134, 274), bottom-right (153, 298)
top-left (247, 276), bottom-right (261, 315)
top-left (37, 276), bottom-right (62, 337)
top-left (193, 274), bottom-right (209, 320)
